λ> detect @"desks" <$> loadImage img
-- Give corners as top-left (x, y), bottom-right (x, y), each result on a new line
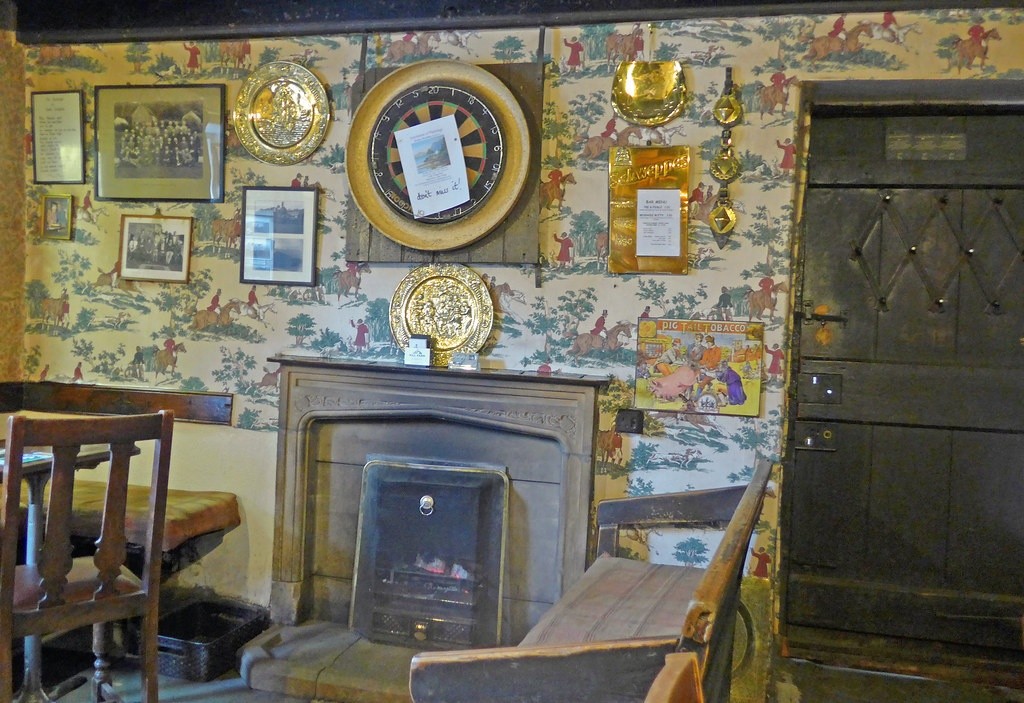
top-left (0, 444), bottom-right (139, 703)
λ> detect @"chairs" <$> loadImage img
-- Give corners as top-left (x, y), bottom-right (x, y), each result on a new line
top-left (0, 407), bottom-right (174, 702)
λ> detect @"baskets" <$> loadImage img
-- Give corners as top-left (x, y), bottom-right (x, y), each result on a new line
top-left (140, 595), bottom-right (269, 681)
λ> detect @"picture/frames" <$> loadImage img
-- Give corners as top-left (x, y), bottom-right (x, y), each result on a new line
top-left (32, 90), bottom-right (86, 185)
top-left (93, 82), bottom-right (227, 203)
top-left (118, 214), bottom-right (193, 284)
top-left (238, 186), bottom-right (319, 287)
top-left (40, 193), bottom-right (72, 240)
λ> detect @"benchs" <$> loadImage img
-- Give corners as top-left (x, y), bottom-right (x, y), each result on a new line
top-left (407, 458), bottom-right (773, 702)
top-left (0, 470), bottom-right (241, 654)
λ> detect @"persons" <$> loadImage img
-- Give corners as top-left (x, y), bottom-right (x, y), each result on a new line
top-left (122, 120), bottom-right (199, 168)
top-left (47, 203), bottom-right (58, 225)
top-left (128, 229), bottom-right (183, 265)
